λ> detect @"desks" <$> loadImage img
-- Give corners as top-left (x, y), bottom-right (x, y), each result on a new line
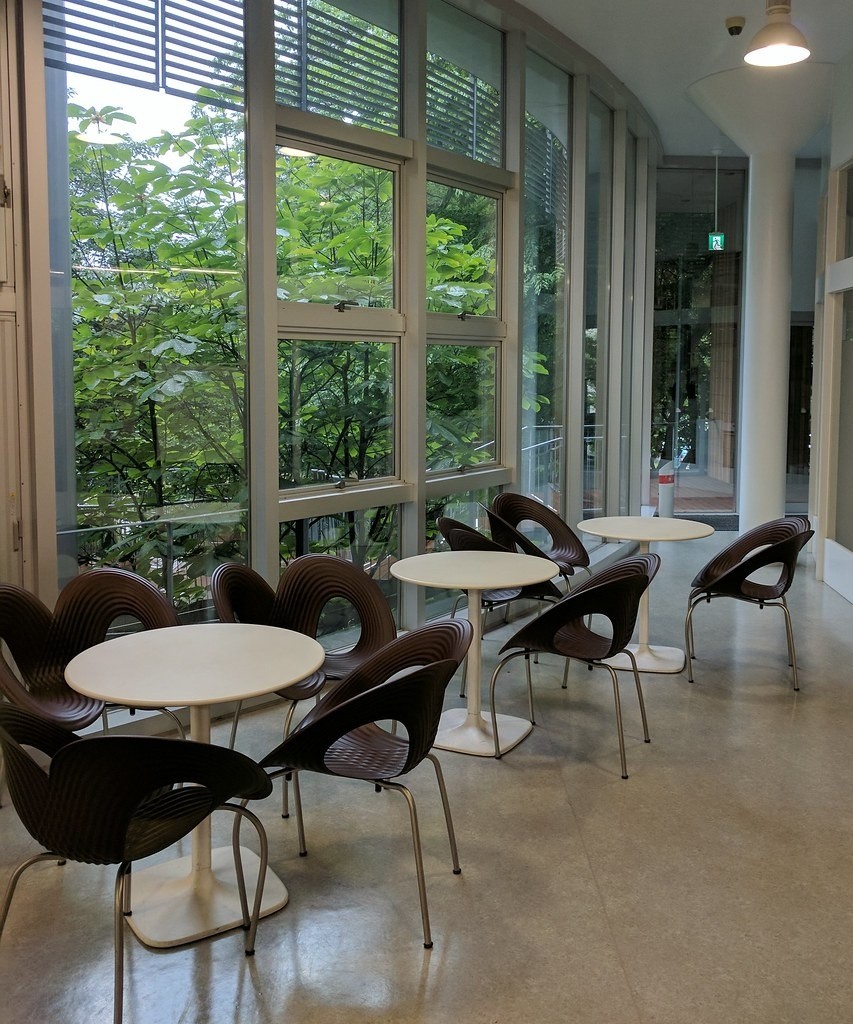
top-left (66, 623), bottom-right (325, 948)
top-left (575, 516), bottom-right (715, 675)
top-left (389, 551), bottom-right (560, 757)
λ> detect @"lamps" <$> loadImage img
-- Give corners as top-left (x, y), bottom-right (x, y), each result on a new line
top-left (708, 150), bottom-right (725, 251)
top-left (743, 1), bottom-right (811, 69)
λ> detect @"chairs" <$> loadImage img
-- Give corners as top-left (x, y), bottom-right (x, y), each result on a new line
top-left (490, 553), bottom-right (661, 778)
top-left (477, 493), bottom-right (595, 671)
top-left (210, 553), bottom-right (397, 818)
top-left (685, 516), bottom-right (814, 693)
top-left (0, 702), bottom-right (272, 1022)
top-left (233, 619), bottom-right (475, 950)
top-left (0, 569), bottom-right (188, 866)
top-left (436, 517), bottom-right (569, 699)
